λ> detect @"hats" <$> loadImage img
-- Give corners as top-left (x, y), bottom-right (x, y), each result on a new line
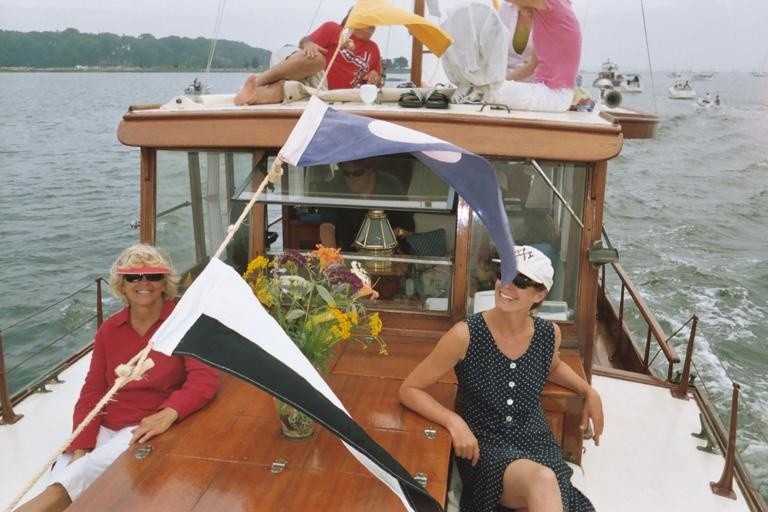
top-left (116, 267), bottom-right (170, 275)
top-left (491, 245), bottom-right (554, 293)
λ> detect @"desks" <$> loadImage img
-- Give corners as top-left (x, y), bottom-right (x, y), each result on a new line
top-left (68, 311), bottom-right (586, 512)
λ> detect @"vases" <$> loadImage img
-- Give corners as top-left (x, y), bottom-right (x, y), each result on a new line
top-left (257, 347), bottom-right (342, 435)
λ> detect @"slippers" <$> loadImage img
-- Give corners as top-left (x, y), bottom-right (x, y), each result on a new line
top-left (399, 91), bottom-right (423, 108)
top-left (425, 90), bottom-right (449, 109)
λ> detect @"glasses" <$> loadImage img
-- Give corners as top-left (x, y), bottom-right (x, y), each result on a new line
top-left (126, 274), bottom-right (161, 282)
top-left (340, 168), bottom-right (365, 178)
top-left (496, 266), bottom-right (543, 289)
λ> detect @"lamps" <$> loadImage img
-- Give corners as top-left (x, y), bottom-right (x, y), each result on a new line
top-left (351, 209), bottom-right (400, 274)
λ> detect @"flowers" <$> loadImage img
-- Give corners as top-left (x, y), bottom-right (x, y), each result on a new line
top-left (234, 243), bottom-right (387, 436)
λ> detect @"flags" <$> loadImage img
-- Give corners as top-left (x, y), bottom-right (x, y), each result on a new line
top-left (342, 0), bottom-right (455, 60)
top-left (146, 253), bottom-right (444, 512)
top-left (277, 93), bottom-right (521, 291)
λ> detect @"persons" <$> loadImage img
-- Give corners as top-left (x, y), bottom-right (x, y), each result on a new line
top-left (713, 95), bottom-right (721, 106)
top-left (479, 1), bottom-right (584, 115)
top-left (11, 239), bottom-right (221, 512)
top-left (192, 77), bottom-right (201, 91)
top-left (702, 92), bottom-right (712, 104)
top-left (317, 158), bottom-right (416, 250)
top-left (230, 2), bottom-right (384, 109)
top-left (395, 242), bottom-right (605, 512)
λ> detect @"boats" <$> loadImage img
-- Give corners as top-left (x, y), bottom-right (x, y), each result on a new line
top-left (665, 71), bottom-right (681, 77)
top-left (593, 78), bottom-right (660, 140)
top-left (0, 1), bottom-right (767, 512)
top-left (690, 72), bottom-right (715, 82)
top-left (185, 84), bottom-right (212, 95)
top-left (696, 97), bottom-right (723, 110)
top-left (591, 58), bottom-right (643, 94)
top-left (668, 83), bottom-right (695, 99)
top-left (749, 65), bottom-right (766, 77)
